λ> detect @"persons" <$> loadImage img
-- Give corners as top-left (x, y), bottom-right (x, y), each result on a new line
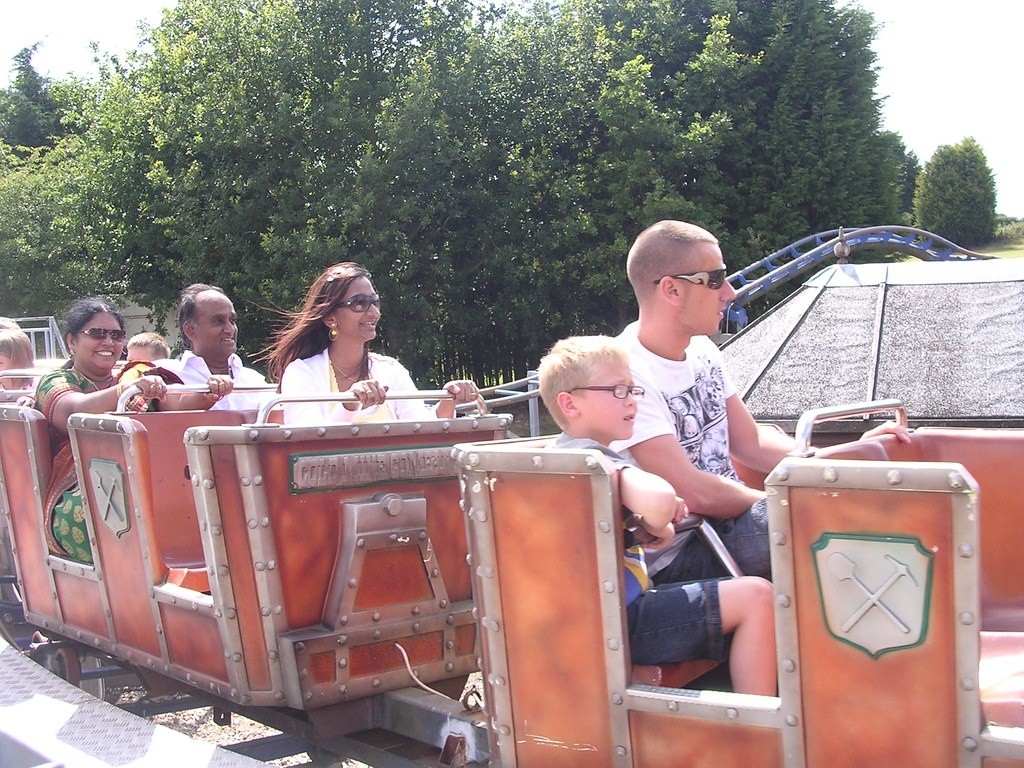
top-left (150, 283), bottom-right (285, 409)
top-left (246, 261), bottom-right (480, 432)
top-left (607, 219), bottom-right (917, 589)
top-left (125, 332), bottom-right (171, 364)
top-left (0, 316), bottom-right (21, 331)
top-left (0, 329), bottom-right (42, 409)
top-left (536, 332), bottom-right (781, 698)
top-left (34, 296), bottom-right (236, 566)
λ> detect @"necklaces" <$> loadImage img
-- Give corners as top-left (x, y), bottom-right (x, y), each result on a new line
top-left (331, 361), bottom-right (363, 382)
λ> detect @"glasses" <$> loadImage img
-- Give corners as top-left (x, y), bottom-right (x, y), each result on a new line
top-left (73, 328), bottom-right (128, 343)
top-left (567, 383), bottom-right (645, 400)
top-left (330, 293), bottom-right (386, 315)
top-left (653, 267), bottom-right (733, 288)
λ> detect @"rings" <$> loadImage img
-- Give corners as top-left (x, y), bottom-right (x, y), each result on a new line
top-left (156, 385), bottom-right (162, 390)
top-left (471, 392), bottom-right (476, 396)
top-left (150, 384), bottom-right (157, 389)
top-left (367, 390), bottom-right (373, 395)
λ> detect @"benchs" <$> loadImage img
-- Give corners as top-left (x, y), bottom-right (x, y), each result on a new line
top-left (810, 427), bottom-right (1024, 736)
top-left (119, 409), bottom-right (293, 594)
